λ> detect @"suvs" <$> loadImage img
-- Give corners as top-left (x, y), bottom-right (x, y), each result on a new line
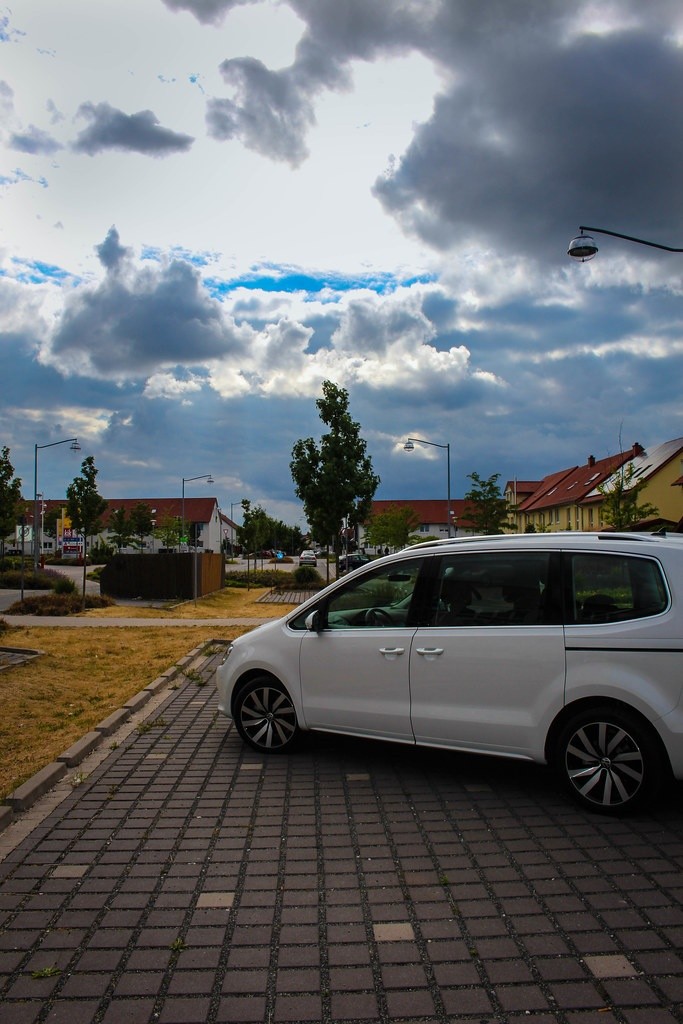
top-left (215, 527), bottom-right (682, 815)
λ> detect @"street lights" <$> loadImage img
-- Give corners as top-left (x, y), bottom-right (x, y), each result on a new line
top-left (150, 519), bottom-right (156, 554)
top-left (182, 474), bottom-right (215, 536)
top-left (404, 437), bottom-right (452, 537)
top-left (34, 437), bottom-right (81, 567)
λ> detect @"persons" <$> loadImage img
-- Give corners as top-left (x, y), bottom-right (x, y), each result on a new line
top-left (385, 546), bottom-right (389, 554)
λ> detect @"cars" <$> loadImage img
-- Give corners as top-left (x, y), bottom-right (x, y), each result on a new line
top-left (250, 548), bottom-right (286, 559)
top-left (298, 550), bottom-right (318, 568)
top-left (337, 554), bottom-right (373, 572)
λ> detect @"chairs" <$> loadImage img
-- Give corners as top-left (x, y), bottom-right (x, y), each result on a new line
top-left (501, 573), bottom-right (541, 611)
top-left (438, 575), bottom-right (480, 626)
